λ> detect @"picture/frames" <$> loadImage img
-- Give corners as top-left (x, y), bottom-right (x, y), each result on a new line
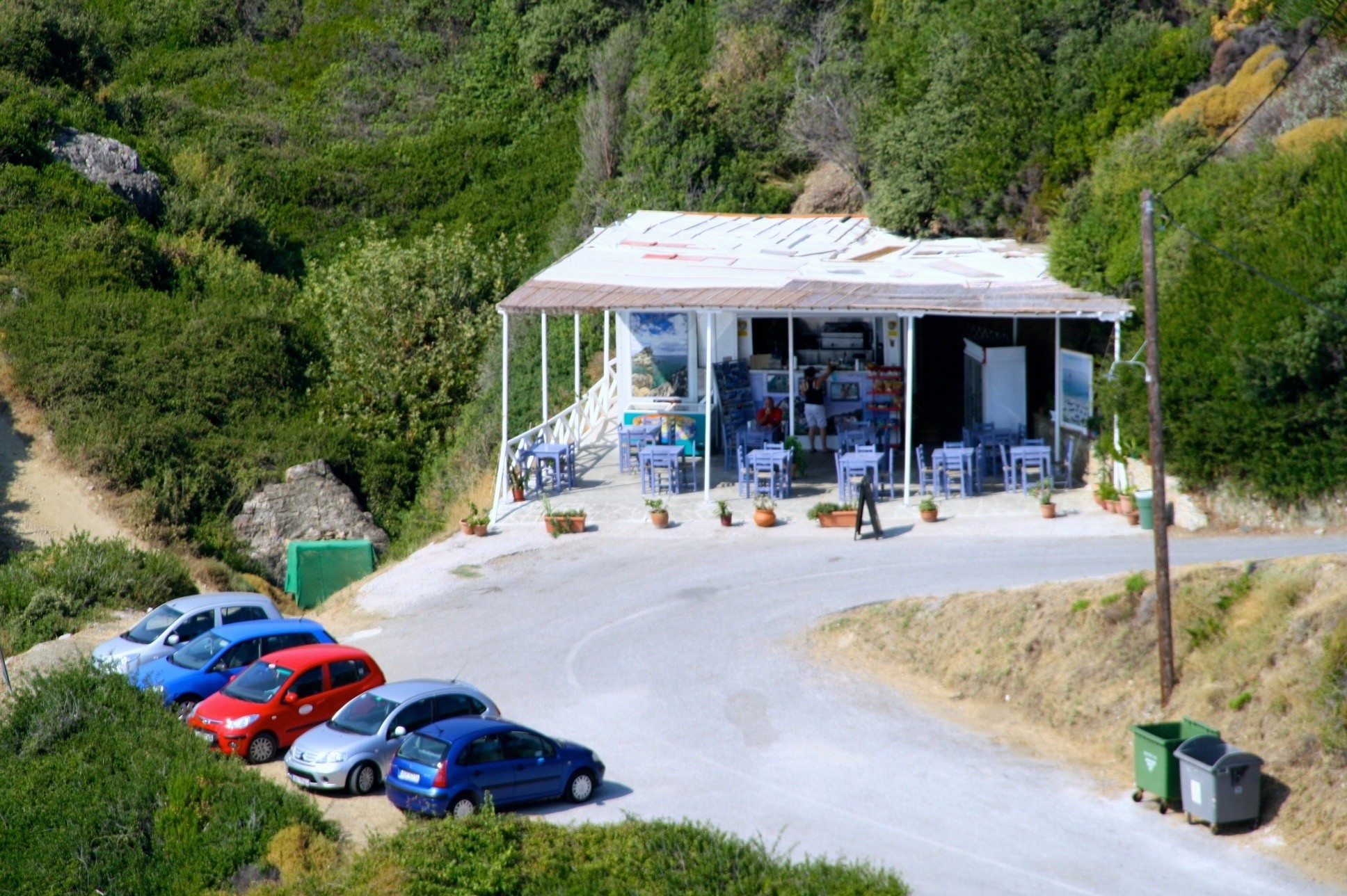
top-left (767, 374), bottom-right (860, 402)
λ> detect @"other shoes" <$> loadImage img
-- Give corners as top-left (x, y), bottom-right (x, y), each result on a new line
top-left (810, 449), bottom-right (818, 453)
top-left (822, 448), bottom-right (832, 453)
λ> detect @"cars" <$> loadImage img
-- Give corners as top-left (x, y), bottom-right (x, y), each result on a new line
top-left (90, 593), bottom-right (282, 676)
top-left (283, 678), bottom-right (501, 796)
top-left (187, 643), bottom-right (388, 764)
top-left (386, 715), bottom-right (604, 819)
top-left (128, 618), bottom-right (336, 721)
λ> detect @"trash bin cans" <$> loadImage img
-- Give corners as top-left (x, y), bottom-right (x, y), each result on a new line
top-left (1130, 718), bottom-right (1223, 815)
top-left (1134, 489), bottom-right (1154, 530)
top-left (1173, 734), bottom-right (1265, 837)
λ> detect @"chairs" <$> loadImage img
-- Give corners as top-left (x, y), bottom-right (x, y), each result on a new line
top-left (517, 418), bottom-right (1075, 500)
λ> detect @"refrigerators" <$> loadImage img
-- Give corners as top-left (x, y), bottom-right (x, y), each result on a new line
top-left (624, 403), bottom-right (717, 456)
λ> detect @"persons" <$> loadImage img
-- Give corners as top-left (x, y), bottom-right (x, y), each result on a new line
top-left (757, 396), bottom-right (783, 443)
top-left (799, 358), bottom-right (834, 453)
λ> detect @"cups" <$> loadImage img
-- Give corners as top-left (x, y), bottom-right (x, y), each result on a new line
top-left (747, 420), bottom-right (752, 428)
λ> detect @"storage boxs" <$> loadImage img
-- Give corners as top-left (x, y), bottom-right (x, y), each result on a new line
top-left (750, 353), bottom-right (772, 370)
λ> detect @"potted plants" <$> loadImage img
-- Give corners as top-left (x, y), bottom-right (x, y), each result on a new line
top-left (540, 491), bottom-right (587, 538)
top-left (548, 456), bottom-right (565, 473)
top-left (784, 434), bottom-right (808, 479)
top-left (507, 466), bottom-right (530, 502)
top-left (642, 495), bottom-right (939, 526)
top-left (1028, 434), bottom-right (1136, 518)
top-left (458, 501), bottom-right (491, 536)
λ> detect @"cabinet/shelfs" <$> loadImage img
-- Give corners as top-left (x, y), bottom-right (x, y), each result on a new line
top-left (866, 376), bottom-right (902, 450)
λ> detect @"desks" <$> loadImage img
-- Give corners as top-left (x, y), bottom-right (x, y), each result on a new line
top-left (745, 449), bottom-right (788, 499)
top-left (524, 443), bottom-right (568, 492)
top-left (930, 447), bottom-right (974, 499)
top-left (736, 425), bottom-right (779, 443)
top-left (638, 444), bottom-right (687, 494)
top-left (983, 426), bottom-right (1012, 446)
top-left (838, 452), bottom-right (886, 501)
top-left (1009, 445), bottom-right (1053, 493)
top-left (618, 425), bottom-right (661, 474)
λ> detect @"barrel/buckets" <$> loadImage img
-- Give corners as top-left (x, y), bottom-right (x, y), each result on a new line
top-left (1134, 496), bottom-right (1153, 530)
top-left (852, 352), bottom-right (866, 371)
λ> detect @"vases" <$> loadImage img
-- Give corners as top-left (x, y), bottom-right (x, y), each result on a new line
top-left (1125, 512), bottom-right (1139, 524)
